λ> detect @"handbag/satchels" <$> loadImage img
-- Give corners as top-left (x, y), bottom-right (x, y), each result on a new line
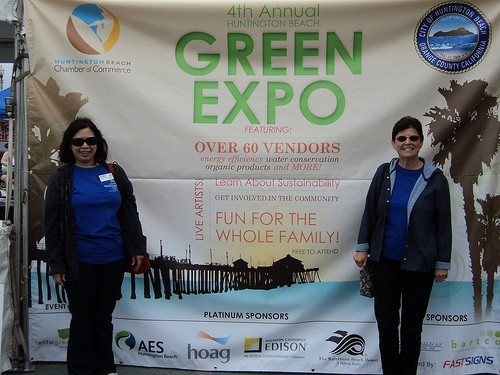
top-left (359, 257), bottom-right (374, 297)
top-left (125, 237), bottom-right (150, 273)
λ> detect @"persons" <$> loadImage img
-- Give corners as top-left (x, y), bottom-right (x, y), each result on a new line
top-left (44, 118), bottom-right (146, 375)
top-left (352, 116), bottom-right (452, 375)
top-left (0, 144), bottom-right (15, 191)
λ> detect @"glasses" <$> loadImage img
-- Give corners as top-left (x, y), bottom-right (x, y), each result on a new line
top-left (396, 135), bottom-right (420, 141)
top-left (72, 136), bottom-right (98, 146)
